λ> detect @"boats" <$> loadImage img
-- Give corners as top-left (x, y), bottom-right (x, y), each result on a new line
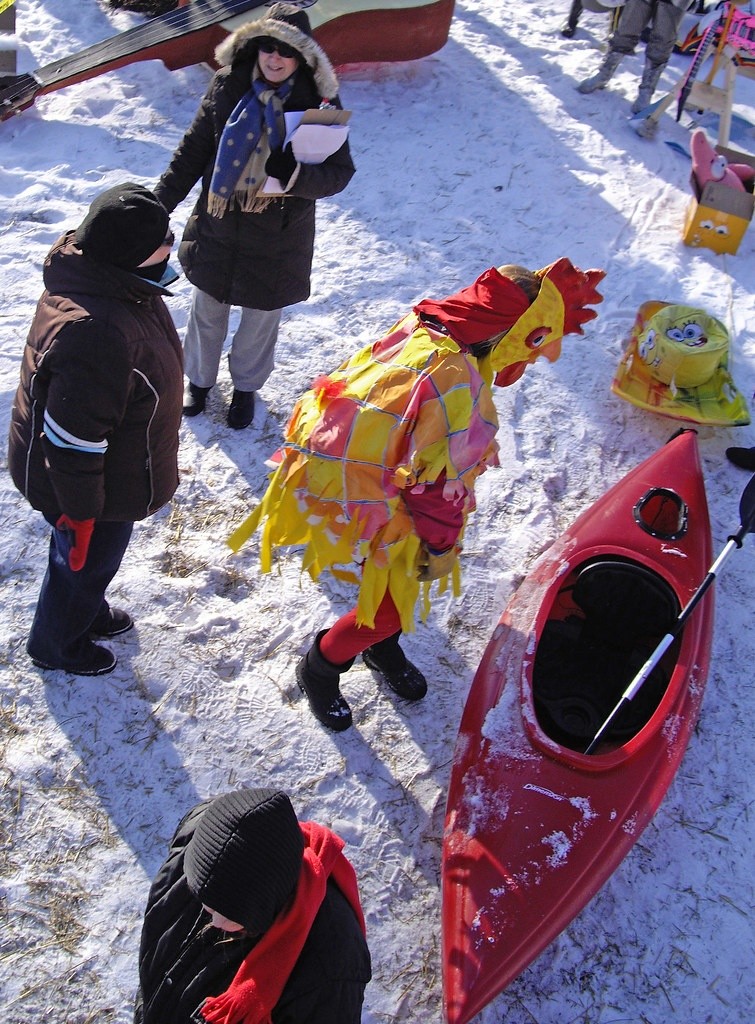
top-left (440, 428), bottom-right (715, 1023)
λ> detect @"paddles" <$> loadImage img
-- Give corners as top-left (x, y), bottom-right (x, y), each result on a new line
top-left (581, 470), bottom-right (755, 757)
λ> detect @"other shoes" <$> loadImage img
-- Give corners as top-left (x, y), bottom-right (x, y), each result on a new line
top-left (726, 447), bottom-right (755, 472)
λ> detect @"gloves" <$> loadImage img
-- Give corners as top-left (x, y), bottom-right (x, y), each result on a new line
top-left (416, 545), bottom-right (456, 584)
top-left (56, 514), bottom-right (96, 571)
top-left (264, 141), bottom-right (296, 189)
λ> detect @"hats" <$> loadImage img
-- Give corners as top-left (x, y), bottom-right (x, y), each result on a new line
top-left (77, 183), bottom-right (170, 265)
top-left (183, 788), bottom-right (305, 936)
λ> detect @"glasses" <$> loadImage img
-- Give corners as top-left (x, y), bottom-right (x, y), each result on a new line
top-left (163, 233), bottom-right (175, 247)
top-left (259, 42), bottom-right (293, 58)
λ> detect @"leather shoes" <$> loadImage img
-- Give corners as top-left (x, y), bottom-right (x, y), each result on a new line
top-left (228, 387), bottom-right (254, 429)
top-left (183, 382), bottom-right (214, 416)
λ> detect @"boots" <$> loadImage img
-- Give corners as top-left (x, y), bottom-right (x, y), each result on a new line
top-left (295, 628), bottom-right (352, 732)
top-left (361, 628), bottom-right (428, 701)
top-left (631, 56), bottom-right (668, 114)
top-left (579, 51), bottom-right (624, 93)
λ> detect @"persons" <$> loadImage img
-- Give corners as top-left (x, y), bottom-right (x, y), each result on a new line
top-left (133, 788), bottom-right (372, 1024)
top-left (225, 256), bottom-right (606, 730)
top-left (7, 183), bottom-right (185, 677)
top-left (153, 3), bottom-right (356, 430)
top-left (561, 0), bottom-right (695, 112)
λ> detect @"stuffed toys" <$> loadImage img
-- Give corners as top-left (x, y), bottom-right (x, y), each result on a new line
top-left (691, 130), bottom-right (755, 193)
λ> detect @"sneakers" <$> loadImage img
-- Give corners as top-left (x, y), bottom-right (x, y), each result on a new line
top-left (33, 641), bottom-right (117, 676)
top-left (82, 607), bottom-right (134, 636)
